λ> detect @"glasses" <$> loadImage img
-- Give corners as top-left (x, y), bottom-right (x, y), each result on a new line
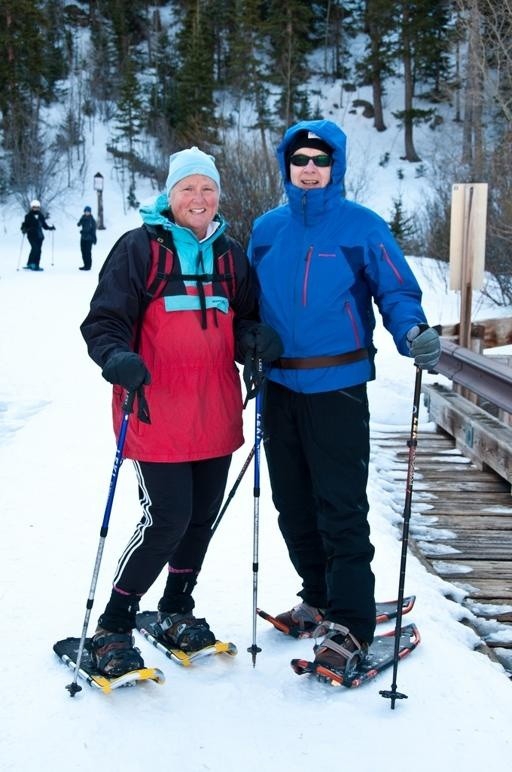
top-left (290, 154), bottom-right (329, 166)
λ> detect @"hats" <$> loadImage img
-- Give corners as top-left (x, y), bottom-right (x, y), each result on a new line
top-left (84, 206), bottom-right (91, 212)
top-left (285, 130), bottom-right (333, 179)
top-left (30, 200), bottom-right (41, 209)
top-left (165, 146), bottom-right (220, 197)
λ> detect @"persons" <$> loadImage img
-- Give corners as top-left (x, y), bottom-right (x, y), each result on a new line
top-left (80, 145), bottom-right (284, 680)
top-left (21, 200), bottom-right (56, 271)
top-left (242, 119), bottom-right (441, 675)
top-left (77, 206), bottom-right (97, 270)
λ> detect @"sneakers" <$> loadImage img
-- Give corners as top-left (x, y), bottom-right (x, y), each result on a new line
top-left (156, 609), bottom-right (215, 652)
top-left (27, 264), bottom-right (43, 271)
top-left (80, 266), bottom-right (90, 270)
top-left (274, 603), bottom-right (368, 670)
top-left (91, 623), bottom-right (144, 678)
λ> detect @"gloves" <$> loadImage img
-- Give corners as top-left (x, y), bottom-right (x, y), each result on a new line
top-left (102, 351), bottom-right (151, 390)
top-left (243, 325), bottom-right (283, 366)
top-left (406, 324), bottom-right (442, 369)
